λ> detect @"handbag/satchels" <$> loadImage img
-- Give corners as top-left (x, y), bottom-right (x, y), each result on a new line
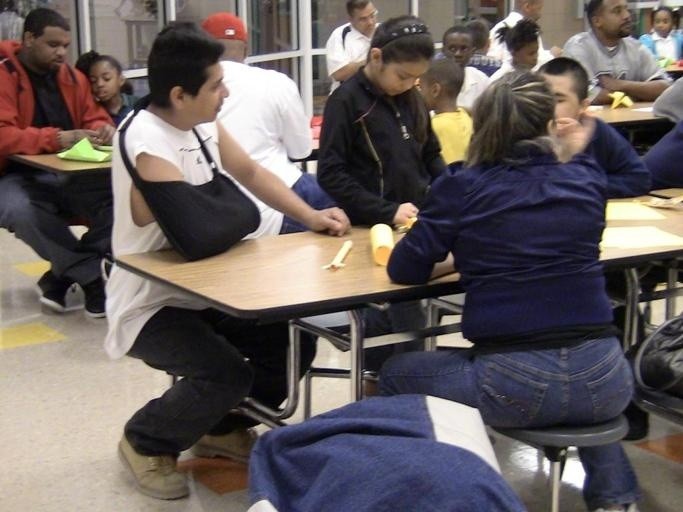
top-left (115, 102), bottom-right (263, 264)
top-left (623, 312), bottom-right (682, 402)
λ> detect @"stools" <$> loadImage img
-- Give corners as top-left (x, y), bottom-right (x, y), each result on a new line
top-left (500, 420), bottom-right (628, 512)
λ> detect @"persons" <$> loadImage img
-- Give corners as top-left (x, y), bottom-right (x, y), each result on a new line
top-left (535, 56), bottom-right (654, 442)
top-left (102, 22), bottom-right (355, 501)
top-left (375, 63), bottom-right (649, 512)
top-left (0, 1), bottom-right (682, 400)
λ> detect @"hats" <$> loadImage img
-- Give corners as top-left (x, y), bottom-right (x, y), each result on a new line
top-left (201, 11), bottom-right (248, 43)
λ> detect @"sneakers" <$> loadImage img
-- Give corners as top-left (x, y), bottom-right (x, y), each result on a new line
top-left (194, 425), bottom-right (261, 465)
top-left (81, 276), bottom-right (110, 319)
top-left (34, 270), bottom-right (66, 313)
top-left (116, 431), bottom-right (191, 501)
